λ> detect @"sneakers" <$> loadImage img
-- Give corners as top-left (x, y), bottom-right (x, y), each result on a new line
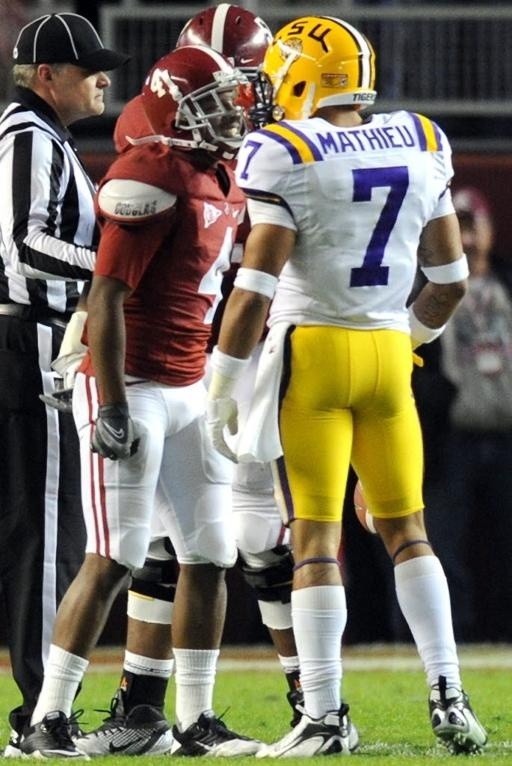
top-left (427, 675), bottom-right (489, 755)
top-left (255, 702), bottom-right (351, 758)
top-left (170, 712), bottom-right (267, 757)
top-left (72, 687), bottom-right (174, 756)
top-left (285, 671), bottom-right (358, 752)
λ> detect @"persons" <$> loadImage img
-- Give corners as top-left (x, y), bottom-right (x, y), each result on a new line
top-left (392, 185), bottom-right (512, 643)
top-left (64, 0), bottom-right (323, 756)
top-left (4, 45), bottom-right (268, 760)
top-left (1, 10), bottom-right (135, 759)
top-left (206, 12), bottom-right (489, 757)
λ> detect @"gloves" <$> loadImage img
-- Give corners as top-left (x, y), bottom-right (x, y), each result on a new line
top-left (89, 403), bottom-right (141, 461)
top-left (206, 345), bottom-right (252, 465)
top-left (408, 302), bottom-right (447, 353)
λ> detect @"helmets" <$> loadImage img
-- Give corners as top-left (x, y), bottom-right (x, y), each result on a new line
top-left (142, 45), bottom-right (256, 162)
top-left (175, 4), bottom-right (274, 77)
top-left (252, 15), bottom-right (377, 124)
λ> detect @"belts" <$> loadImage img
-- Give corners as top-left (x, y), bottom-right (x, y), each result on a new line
top-left (0, 304), bottom-right (67, 329)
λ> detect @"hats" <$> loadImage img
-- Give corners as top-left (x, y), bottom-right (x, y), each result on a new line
top-left (12, 12), bottom-right (134, 72)
top-left (452, 187), bottom-right (488, 214)
